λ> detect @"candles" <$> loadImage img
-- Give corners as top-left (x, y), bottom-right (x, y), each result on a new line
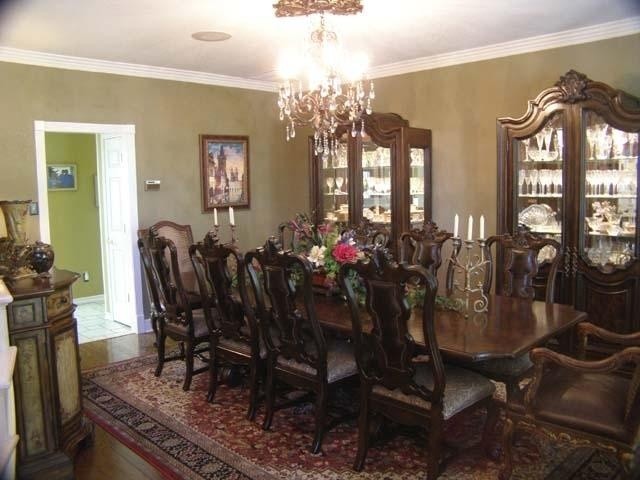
top-left (227, 204), bottom-right (238, 241)
top-left (209, 205), bottom-right (222, 236)
top-left (477, 211), bottom-right (487, 260)
top-left (452, 212), bottom-right (461, 264)
top-left (466, 212), bottom-right (475, 257)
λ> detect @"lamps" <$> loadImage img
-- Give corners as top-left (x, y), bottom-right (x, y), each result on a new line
top-left (274, 1), bottom-right (377, 160)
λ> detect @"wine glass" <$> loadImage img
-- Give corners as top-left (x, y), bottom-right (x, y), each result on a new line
top-left (539, 234), bottom-right (562, 263)
top-left (363, 147), bottom-right (390, 168)
top-left (521, 128), bottom-right (564, 163)
top-left (410, 204), bottom-right (425, 223)
top-left (336, 177), bottom-right (344, 194)
top-left (410, 177), bottom-right (424, 194)
top-left (585, 201), bottom-right (636, 235)
top-left (518, 169), bottom-right (562, 197)
top-left (586, 239), bottom-right (636, 268)
top-left (327, 205), bottom-right (349, 221)
top-left (326, 177), bottom-right (334, 195)
top-left (410, 149), bottom-right (424, 166)
top-left (363, 171), bottom-right (391, 194)
top-left (586, 169), bottom-right (636, 195)
top-left (362, 207), bottom-right (392, 224)
top-left (585, 123), bottom-right (638, 158)
top-left (322, 144), bottom-right (346, 168)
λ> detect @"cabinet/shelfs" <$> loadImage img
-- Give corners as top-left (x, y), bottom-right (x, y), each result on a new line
top-left (494, 70), bottom-right (640, 377)
top-left (307, 111), bottom-right (441, 259)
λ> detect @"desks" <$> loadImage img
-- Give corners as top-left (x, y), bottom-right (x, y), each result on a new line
top-left (216, 266), bottom-right (587, 462)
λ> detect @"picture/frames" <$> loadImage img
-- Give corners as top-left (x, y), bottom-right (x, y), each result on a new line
top-left (200, 133), bottom-right (253, 211)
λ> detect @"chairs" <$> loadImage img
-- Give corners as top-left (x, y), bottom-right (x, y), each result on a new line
top-left (137, 228), bottom-right (224, 396)
top-left (189, 229), bottom-right (270, 421)
top-left (341, 217), bottom-right (392, 249)
top-left (139, 220), bottom-right (204, 350)
top-left (275, 209), bottom-right (316, 256)
top-left (484, 225), bottom-right (559, 305)
top-left (485, 317), bottom-right (640, 478)
top-left (401, 219), bottom-right (459, 296)
top-left (245, 237), bottom-right (362, 453)
top-left (339, 259), bottom-right (503, 479)
